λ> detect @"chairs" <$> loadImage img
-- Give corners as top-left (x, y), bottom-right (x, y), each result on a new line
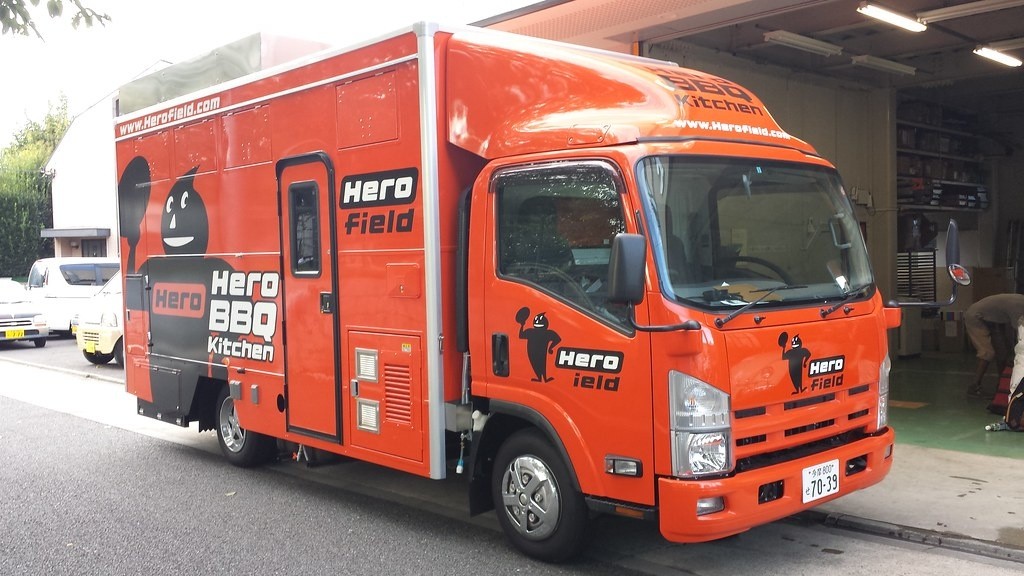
top-left (504, 196), bottom-right (582, 298)
top-left (665, 205), bottom-right (690, 287)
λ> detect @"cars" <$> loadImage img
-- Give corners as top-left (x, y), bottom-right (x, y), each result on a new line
top-left (0, 278), bottom-right (51, 349)
top-left (76, 270), bottom-right (126, 369)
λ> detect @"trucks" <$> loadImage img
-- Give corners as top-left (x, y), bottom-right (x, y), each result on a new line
top-left (109, 18), bottom-right (972, 565)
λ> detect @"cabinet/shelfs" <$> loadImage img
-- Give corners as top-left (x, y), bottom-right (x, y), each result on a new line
top-left (896, 103), bottom-right (989, 211)
top-left (648, 45), bottom-right (892, 361)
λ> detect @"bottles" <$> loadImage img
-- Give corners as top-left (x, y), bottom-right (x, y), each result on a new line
top-left (985, 421), bottom-right (1006, 431)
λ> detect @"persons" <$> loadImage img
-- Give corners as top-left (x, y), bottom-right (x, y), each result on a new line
top-left (964, 294), bottom-right (1024, 398)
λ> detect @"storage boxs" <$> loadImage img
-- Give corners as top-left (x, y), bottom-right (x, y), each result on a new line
top-left (920, 266), bottom-right (1014, 354)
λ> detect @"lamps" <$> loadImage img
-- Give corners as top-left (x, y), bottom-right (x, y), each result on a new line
top-left (851, 55), bottom-right (917, 77)
top-left (973, 36), bottom-right (1024, 67)
top-left (916, 0), bottom-right (1024, 25)
top-left (764, 29), bottom-right (843, 57)
top-left (855, 0), bottom-right (927, 32)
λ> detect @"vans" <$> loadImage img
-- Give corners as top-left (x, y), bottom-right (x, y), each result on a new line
top-left (27, 256), bottom-right (122, 341)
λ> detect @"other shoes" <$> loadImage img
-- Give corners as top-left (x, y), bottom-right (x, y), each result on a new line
top-left (965, 383), bottom-right (993, 399)
